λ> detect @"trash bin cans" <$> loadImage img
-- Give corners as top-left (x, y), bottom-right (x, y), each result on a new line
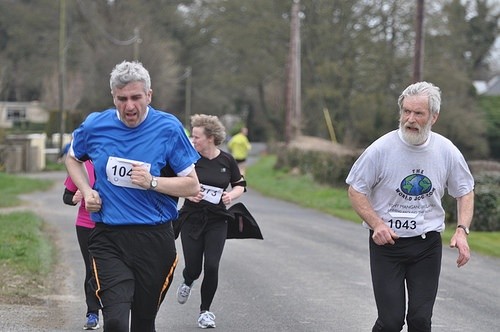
top-left (25, 134), bottom-right (47, 171)
top-left (5, 134), bottom-right (25, 172)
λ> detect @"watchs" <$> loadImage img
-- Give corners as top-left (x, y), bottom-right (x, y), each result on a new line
top-left (147, 175), bottom-right (158, 191)
top-left (457, 225), bottom-right (470, 235)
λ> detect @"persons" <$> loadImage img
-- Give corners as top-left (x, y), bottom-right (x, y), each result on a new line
top-left (65, 60), bottom-right (200, 332)
top-left (227, 127), bottom-right (253, 178)
top-left (63, 160), bottom-right (100, 330)
top-left (345, 82), bottom-right (475, 332)
top-left (173, 114), bottom-right (264, 329)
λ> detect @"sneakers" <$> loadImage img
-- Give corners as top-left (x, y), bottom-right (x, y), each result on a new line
top-left (177, 281), bottom-right (193, 304)
top-left (198, 311), bottom-right (216, 328)
top-left (83, 314), bottom-right (100, 329)
